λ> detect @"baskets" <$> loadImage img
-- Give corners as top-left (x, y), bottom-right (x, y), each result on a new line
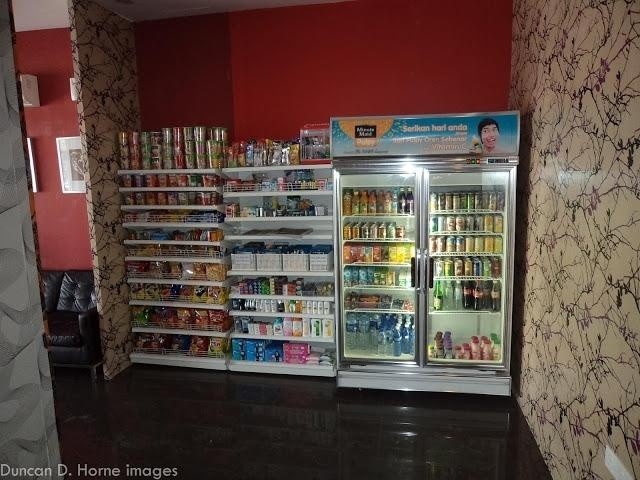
top-left (230, 253), bottom-right (334, 271)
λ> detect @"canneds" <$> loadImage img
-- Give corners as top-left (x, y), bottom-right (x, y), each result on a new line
top-left (344, 221), bottom-right (406, 240)
top-left (432, 256), bottom-right (502, 276)
top-left (431, 215), bottom-right (504, 234)
top-left (430, 191), bottom-right (505, 211)
top-left (118, 127), bottom-right (223, 170)
top-left (429, 236), bottom-right (504, 254)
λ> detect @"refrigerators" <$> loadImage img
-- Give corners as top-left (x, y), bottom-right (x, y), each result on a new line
top-left (329, 110), bottom-right (522, 400)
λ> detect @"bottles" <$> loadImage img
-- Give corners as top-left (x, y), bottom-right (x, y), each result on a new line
top-left (427, 189), bottom-right (504, 362)
top-left (230, 301), bottom-right (333, 338)
top-left (341, 187), bottom-right (415, 356)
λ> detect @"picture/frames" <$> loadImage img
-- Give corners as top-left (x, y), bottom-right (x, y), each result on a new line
top-left (56, 131), bottom-right (89, 194)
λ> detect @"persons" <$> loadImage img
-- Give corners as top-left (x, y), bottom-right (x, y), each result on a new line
top-left (469, 118), bottom-right (507, 154)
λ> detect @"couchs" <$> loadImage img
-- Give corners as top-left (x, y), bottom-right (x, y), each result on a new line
top-left (37, 268), bottom-right (103, 379)
